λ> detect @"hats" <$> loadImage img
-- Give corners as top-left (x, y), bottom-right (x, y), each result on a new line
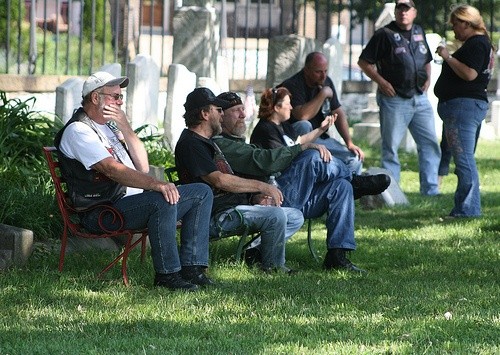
top-left (82, 72), bottom-right (130, 97)
top-left (396, 0), bottom-right (415, 8)
top-left (186, 87), bottom-right (230, 108)
top-left (215, 91), bottom-right (242, 109)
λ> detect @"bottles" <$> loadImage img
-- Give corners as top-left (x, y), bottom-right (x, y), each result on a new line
top-left (321, 97), bottom-right (331, 118)
top-left (434, 37), bottom-right (446, 64)
top-left (267, 175), bottom-right (278, 204)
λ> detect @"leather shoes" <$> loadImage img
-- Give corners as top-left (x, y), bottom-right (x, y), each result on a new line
top-left (181, 266), bottom-right (213, 286)
top-left (322, 252), bottom-right (366, 273)
top-left (350, 174), bottom-right (391, 200)
top-left (153, 274), bottom-right (196, 291)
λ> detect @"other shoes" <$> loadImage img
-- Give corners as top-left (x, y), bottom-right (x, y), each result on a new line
top-left (245, 251), bottom-right (260, 266)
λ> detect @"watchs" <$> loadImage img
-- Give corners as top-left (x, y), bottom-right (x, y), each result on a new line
top-left (445, 55), bottom-right (453, 61)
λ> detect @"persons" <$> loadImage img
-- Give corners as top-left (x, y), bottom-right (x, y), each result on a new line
top-left (214, 92), bottom-right (392, 275)
top-left (274, 52), bottom-right (366, 175)
top-left (434, 5), bottom-right (492, 218)
top-left (249, 87), bottom-right (339, 149)
top-left (357, 0), bottom-right (442, 197)
top-left (173, 87), bottom-right (305, 276)
top-left (434, 16), bottom-right (495, 177)
top-left (55, 71), bottom-right (217, 289)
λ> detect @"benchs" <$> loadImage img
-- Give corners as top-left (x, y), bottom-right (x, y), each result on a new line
top-left (164, 166), bottom-right (319, 263)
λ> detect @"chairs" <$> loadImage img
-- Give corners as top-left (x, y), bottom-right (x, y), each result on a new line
top-left (44, 147), bottom-right (150, 288)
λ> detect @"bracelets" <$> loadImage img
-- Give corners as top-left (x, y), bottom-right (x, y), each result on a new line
top-left (316, 129), bottom-right (322, 137)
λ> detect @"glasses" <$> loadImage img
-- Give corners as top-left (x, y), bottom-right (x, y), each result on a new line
top-left (271, 87), bottom-right (279, 108)
top-left (205, 107), bottom-right (222, 114)
top-left (97, 92), bottom-right (124, 99)
